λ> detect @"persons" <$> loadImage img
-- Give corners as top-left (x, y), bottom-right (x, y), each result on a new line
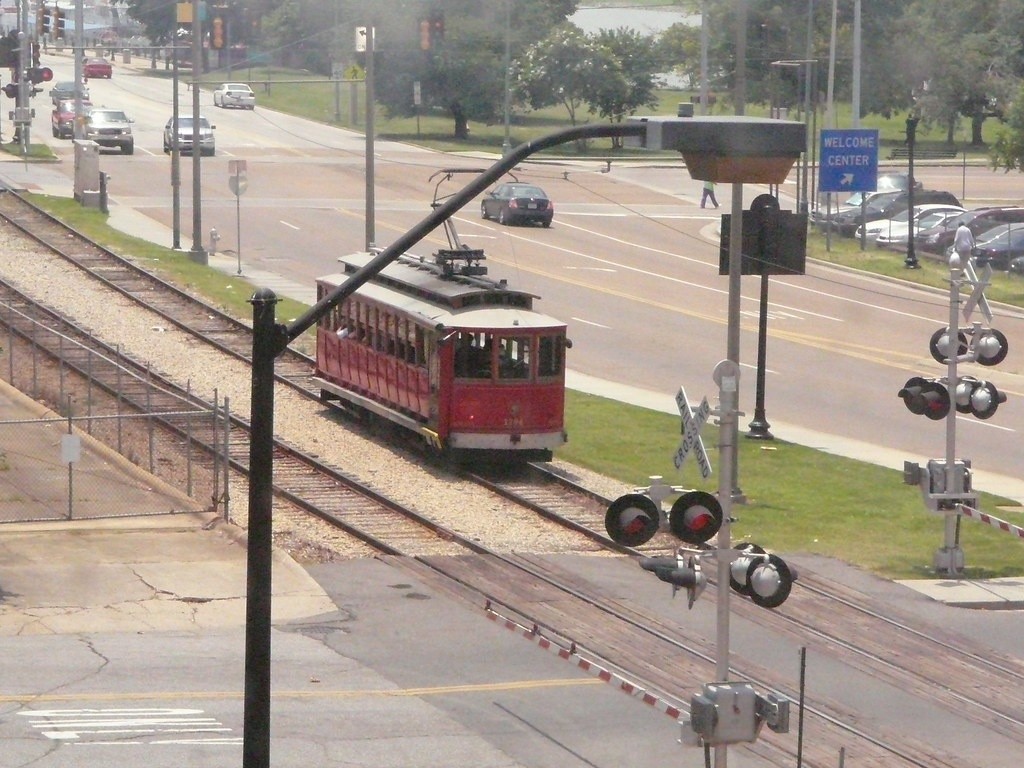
top-left (454, 335), bottom-right (509, 378)
top-left (336, 319), bottom-right (370, 345)
top-left (953, 222), bottom-right (974, 276)
top-left (700, 181), bottom-right (722, 208)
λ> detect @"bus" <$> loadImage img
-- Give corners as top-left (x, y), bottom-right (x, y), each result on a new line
top-left (312, 166), bottom-right (573, 468)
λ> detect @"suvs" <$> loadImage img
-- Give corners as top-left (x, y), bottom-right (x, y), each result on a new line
top-left (832, 190), bottom-right (965, 239)
top-left (82, 107), bottom-right (135, 155)
top-left (917, 206), bottom-right (1024, 256)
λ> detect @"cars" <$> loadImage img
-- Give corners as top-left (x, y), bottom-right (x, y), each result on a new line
top-left (874, 211), bottom-right (965, 248)
top-left (480, 181), bottom-right (553, 227)
top-left (945, 223), bottom-right (1024, 269)
top-left (52, 99), bottom-right (94, 138)
top-left (49, 81), bottom-right (90, 105)
top-left (163, 115), bottom-right (216, 155)
top-left (82, 57), bottom-right (112, 80)
top-left (808, 174), bottom-right (925, 232)
top-left (213, 82), bottom-right (255, 110)
top-left (855, 204), bottom-right (968, 246)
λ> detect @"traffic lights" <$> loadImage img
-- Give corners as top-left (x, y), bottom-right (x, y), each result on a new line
top-left (917, 383), bottom-right (952, 420)
top-left (669, 492), bottom-right (708, 545)
top-left (605, 495), bottom-right (658, 546)
top-left (51, 8), bottom-right (66, 41)
top-left (37, 7), bottom-right (51, 34)
top-left (40, 68), bottom-right (53, 82)
top-left (897, 376), bottom-right (929, 415)
top-left (428, 8), bottom-right (444, 43)
